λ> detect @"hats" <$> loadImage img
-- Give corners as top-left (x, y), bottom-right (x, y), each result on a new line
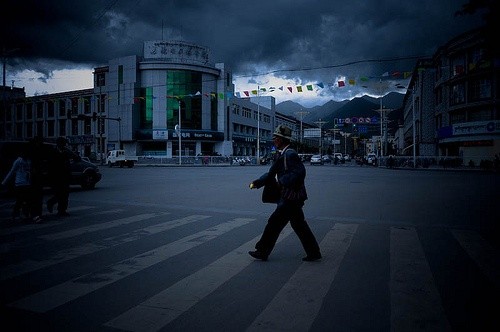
top-left (272, 125), bottom-right (294, 140)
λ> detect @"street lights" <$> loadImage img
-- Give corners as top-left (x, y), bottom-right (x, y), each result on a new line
top-left (361, 78), bottom-right (405, 156)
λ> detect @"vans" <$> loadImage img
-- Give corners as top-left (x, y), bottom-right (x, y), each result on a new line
top-left (0, 140), bottom-right (102, 190)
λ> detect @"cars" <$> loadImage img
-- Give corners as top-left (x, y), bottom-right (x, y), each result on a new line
top-left (310, 155), bottom-right (324, 165)
top-left (321, 152), bottom-right (375, 165)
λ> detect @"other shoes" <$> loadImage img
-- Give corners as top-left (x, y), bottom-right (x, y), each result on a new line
top-left (248, 250), bottom-right (268, 262)
top-left (302, 255), bottom-right (322, 261)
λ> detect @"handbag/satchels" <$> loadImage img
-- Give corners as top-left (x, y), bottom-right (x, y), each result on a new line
top-left (262, 182), bottom-right (280, 204)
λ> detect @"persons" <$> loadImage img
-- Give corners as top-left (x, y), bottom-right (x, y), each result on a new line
top-left (46, 137), bottom-right (74, 217)
top-left (248, 125), bottom-right (322, 261)
top-left (0, 149), bottom-right (43, 224)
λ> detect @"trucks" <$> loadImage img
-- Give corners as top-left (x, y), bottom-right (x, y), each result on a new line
top-left (105, 150), bottom-right (138, 168)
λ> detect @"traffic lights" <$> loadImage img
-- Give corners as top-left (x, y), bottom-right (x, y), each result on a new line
top-left (92, 112), bottom-right (98, 122)
